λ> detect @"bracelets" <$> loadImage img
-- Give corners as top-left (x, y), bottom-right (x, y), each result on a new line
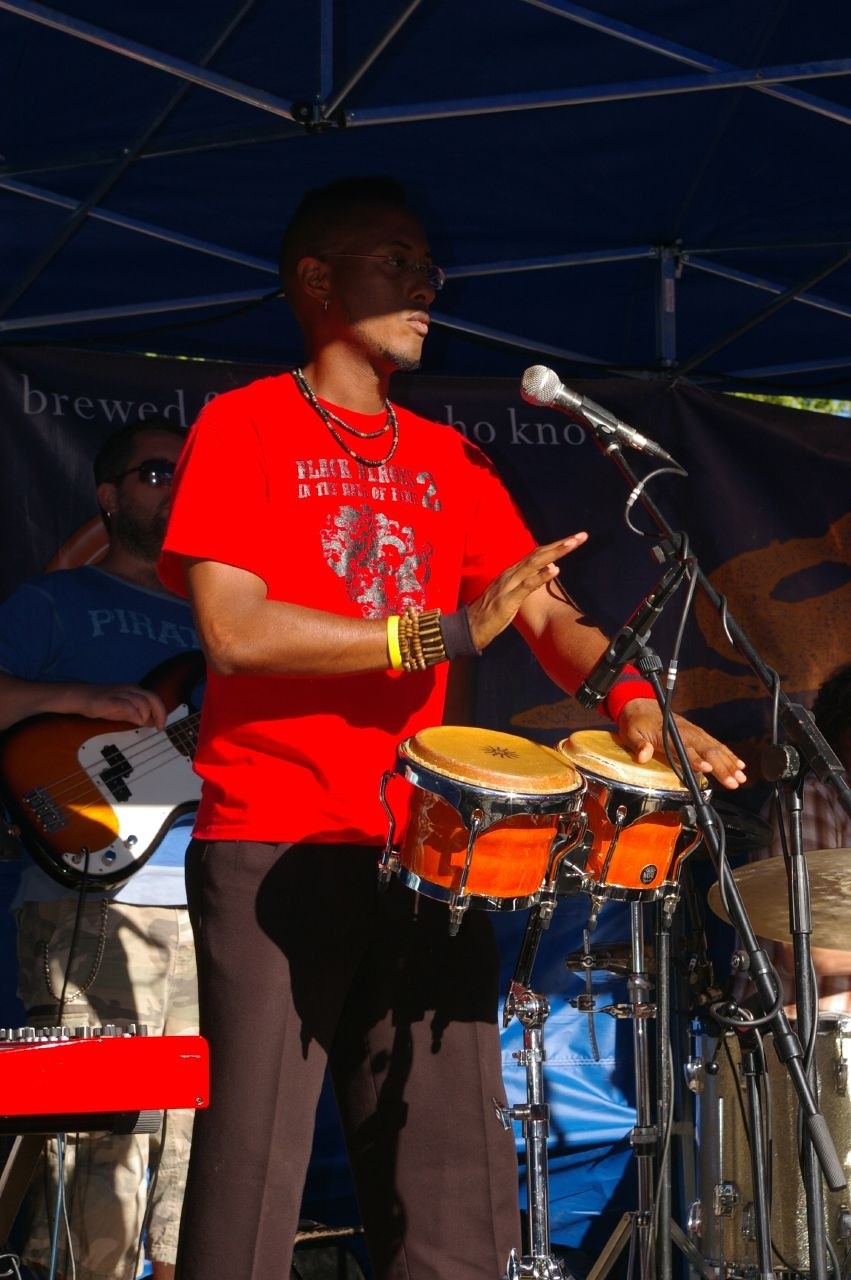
top-left (384, 615), bottom-right (402, 672)
top-left (398, 604), bottom-right (427, 674)
top-left (418, 605), bottom-right (448, 667)
top-left (439, 602), bottom-right (483, 664)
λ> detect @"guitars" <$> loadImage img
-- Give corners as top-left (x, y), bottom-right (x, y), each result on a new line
top-left (2, 649), bottom-right (219, 890)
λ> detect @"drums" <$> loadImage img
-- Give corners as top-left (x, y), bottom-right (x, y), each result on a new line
top-left (384, 717), bottom-right (585, 904)
top-left (557, 727), bottom-right (690, 882)
top-left (697, 1011), bottom-right (851, 1261)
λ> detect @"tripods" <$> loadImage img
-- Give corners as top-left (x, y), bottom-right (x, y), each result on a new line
top-left (586, 903), bottom-right (720, 1280)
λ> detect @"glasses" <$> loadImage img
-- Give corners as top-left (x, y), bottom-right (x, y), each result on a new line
top-left (111, 457), bottom-right (179, 487)
top-left (316, 251), bottom-right (447, 292)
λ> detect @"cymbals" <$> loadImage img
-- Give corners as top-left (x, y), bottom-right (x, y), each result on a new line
top-left (702, 848), bottom-right (851, 953)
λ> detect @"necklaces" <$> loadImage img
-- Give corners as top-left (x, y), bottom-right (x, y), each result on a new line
top-left (289, 364), bottom-right (400, 468)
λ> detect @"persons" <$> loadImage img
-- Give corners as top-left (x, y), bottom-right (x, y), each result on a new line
top-left (0, 419), bottom-right (206, 1279)
top-left (152, 172), bottom-right (751, 1280)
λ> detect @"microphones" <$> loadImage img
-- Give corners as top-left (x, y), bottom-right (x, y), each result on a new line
top-left (519, 364), bottom-right (670, 463)
top-left (575, 558), bottom-right (686, 709)
